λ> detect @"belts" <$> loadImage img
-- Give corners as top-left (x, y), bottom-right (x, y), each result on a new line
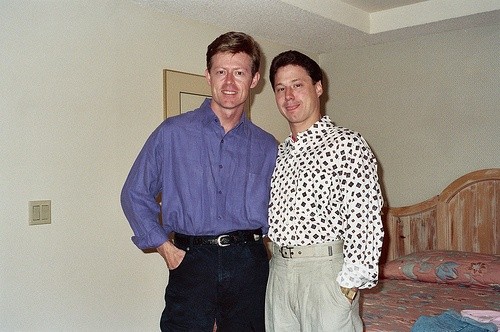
top-left (273, 242), bottom-right (344, 259)
top-left (174, 229), bottom-right (262, 246)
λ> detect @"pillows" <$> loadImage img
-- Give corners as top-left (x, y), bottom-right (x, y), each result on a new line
top-left (378, 250), bottom-right (500, 286)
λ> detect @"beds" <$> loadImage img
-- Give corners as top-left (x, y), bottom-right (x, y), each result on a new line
top-left (359, 169), bottom-right (500, 332)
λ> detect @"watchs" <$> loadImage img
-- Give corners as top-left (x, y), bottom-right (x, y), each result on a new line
top-left (339, 287), bottom-right (356, 302)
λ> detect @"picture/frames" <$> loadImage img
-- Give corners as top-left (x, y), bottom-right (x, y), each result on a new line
top-left (163, 69), bottom-right (250, 122)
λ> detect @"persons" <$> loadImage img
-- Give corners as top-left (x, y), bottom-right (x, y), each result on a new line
top-left (264, 50), bottom-right (385, 332)
top-left (121, 31), bottom-right (278, 332)
